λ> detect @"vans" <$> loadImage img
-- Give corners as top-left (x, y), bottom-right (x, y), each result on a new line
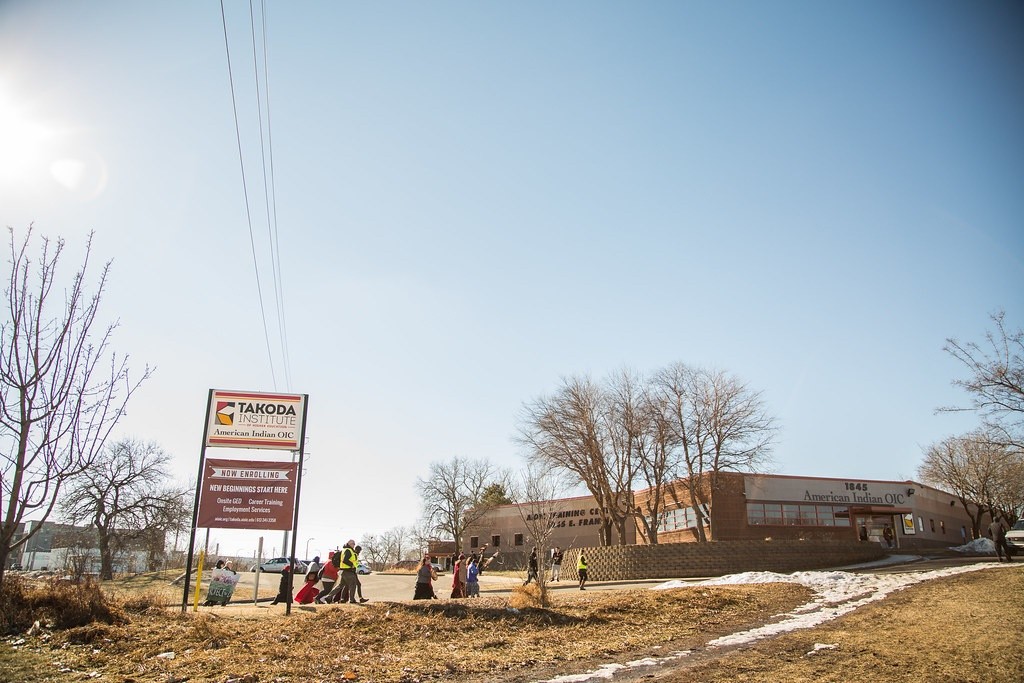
top-left (1004, 518), bottom-right (1024, 555)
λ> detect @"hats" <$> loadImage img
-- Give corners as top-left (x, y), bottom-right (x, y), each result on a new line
top-left (284, 565), bottom-right (291, 571)
top-left (329, 552), bottom-right (334, 558)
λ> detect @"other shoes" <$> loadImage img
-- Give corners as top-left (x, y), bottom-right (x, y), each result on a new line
top-left (314, 598), bottom-right (369, 604)
top-left (580, 586), bottom-right (586, 590)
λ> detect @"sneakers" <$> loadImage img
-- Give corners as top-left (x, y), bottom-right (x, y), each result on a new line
top-left (550, 578), bottom-right (554, 582)
top-left (557, 578), bottom-right (559, 582)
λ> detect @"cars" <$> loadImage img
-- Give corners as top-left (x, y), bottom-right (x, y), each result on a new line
top-left (431, 562), bottom-right (444, 572)
top-left (250, 556), bottom-right (324, 574)
top-left (356, 564), bottom-right (372, 575)
top-left (390, 558), bottom-right (421, 571)
top-left (10, 562), bottom-right (23, 571)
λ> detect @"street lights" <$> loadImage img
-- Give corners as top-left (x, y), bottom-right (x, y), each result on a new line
top-left (305, 537), bottom-right (315, 561)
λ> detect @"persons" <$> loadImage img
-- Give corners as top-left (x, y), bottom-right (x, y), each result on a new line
top-left (294, 539), bottom-right (369, 604)
top-left (414, 555), bottom-right (438, 600)
top-left (522, 547), bottom-right (539, 586)
top-left (577, 548), bottom-right (587, 590)
top-left (883, 524), bottom-right (893, 547)
top-left (987, 517), bottom-right (1013, 562)
top-left (859, 525), bottom-right (868, 541)
top-left (270, 566), bottom-right (293, 605)
top-left (203, 560), bottom-right (240, 607)
top-left (446, 550), bottom-right (484, 598)
top-left (550, 547), bottom-right (563, 582)
